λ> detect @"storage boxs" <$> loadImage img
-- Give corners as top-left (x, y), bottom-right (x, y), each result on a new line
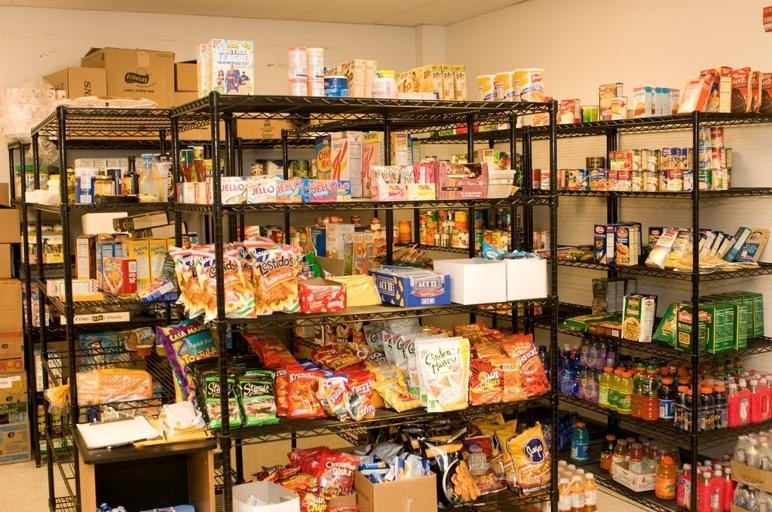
top-left (0, 180), bottom-right (31, 467)
top-left (42, 45), bottom-right (311, 140)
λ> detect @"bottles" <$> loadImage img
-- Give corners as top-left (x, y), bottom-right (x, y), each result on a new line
top-left (566, 419), bottom-right (772, 512)
top-left (538, 337), bottom-right (772, 432)
